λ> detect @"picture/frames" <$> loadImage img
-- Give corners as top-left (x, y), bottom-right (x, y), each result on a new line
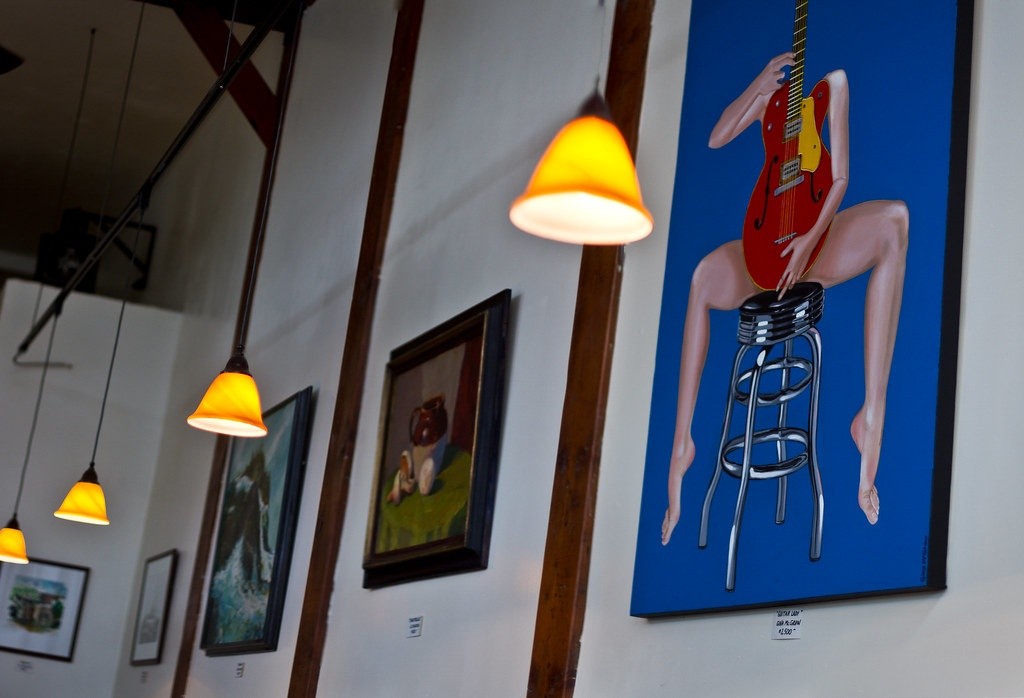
top-left (1, 556), bottom-right (91, 661)
top-left (359, 284), bottom-right (512, 589)
top-left (128, 547), bottom-right (178, 666)
top-left (196, 385), bottom-right (311, 658)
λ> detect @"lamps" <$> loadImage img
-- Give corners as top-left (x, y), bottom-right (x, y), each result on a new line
top-left (54, 172), bottom-right (162, 526)
top-left (506, 3), bottom-right (653, 246)
top-left (186, 6), bottom-right (305, 438)
top-left (0, 303), bottom-right (63, 565)
